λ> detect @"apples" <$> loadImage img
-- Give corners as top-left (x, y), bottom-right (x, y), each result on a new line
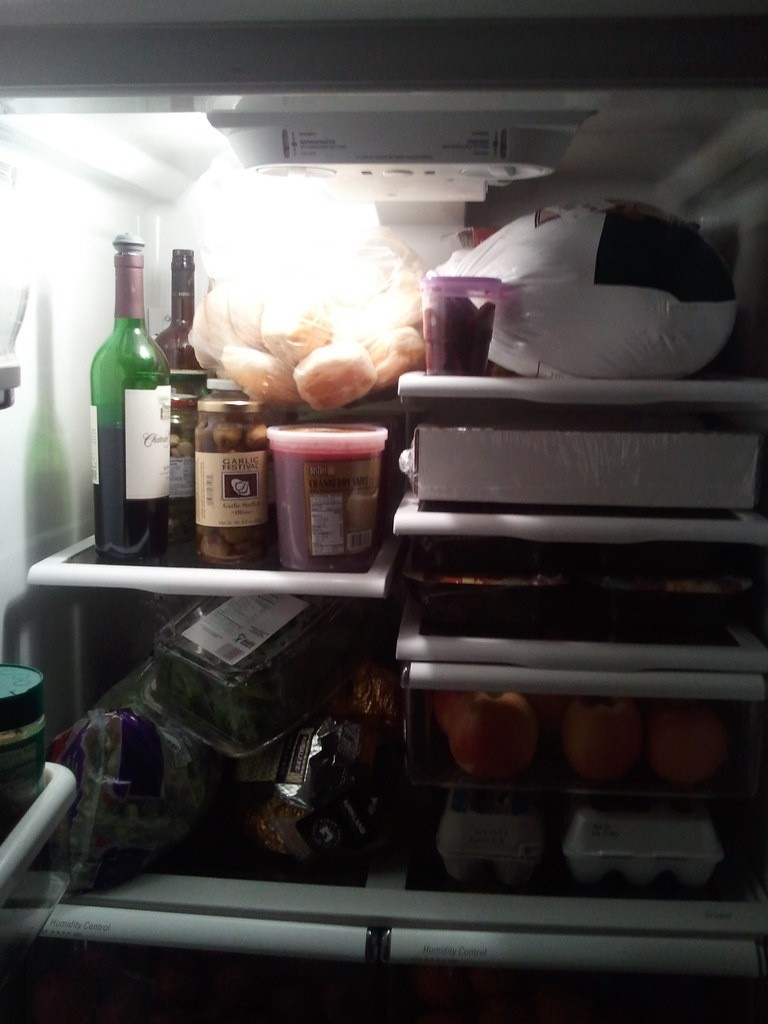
top-left (430, 690), bottom-right (727, 783)
top-left (24, 941), bottom-right (601, 1024)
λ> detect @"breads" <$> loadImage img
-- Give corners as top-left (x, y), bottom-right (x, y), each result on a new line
top-left (249, 658), bottom-right (404, 855)
top-left (192, 277), bottom-right (425, 410)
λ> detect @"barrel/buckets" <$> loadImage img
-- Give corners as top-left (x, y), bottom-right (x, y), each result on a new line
top-left (265, 422), bottom-right (388, 571)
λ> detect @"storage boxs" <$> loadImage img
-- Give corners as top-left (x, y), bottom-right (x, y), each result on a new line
top-left (136, 595), bottom-right (380, 757)
top-left (407, 413), bottom-right (763, 515)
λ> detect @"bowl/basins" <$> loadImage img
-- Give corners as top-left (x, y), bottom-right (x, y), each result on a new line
top-left (440, 792), bottom-right (725, 891)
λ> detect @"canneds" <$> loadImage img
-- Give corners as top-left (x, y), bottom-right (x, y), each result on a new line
top-left (169, 368), bottom-right (270, 562)
top-left (0, 663), bottom-right (45, 844)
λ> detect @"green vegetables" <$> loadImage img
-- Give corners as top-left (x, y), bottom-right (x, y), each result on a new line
top-left (154, 592), bottom-right (364, 744)
top-left (55, 670), bottom-right (221, 891)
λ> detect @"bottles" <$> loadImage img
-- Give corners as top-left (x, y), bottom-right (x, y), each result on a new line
top-left (90, 231), bottom-right (217, 567)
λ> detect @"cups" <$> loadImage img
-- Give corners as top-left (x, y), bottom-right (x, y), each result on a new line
top-left (420, 275), bottom-right (501, 376)
